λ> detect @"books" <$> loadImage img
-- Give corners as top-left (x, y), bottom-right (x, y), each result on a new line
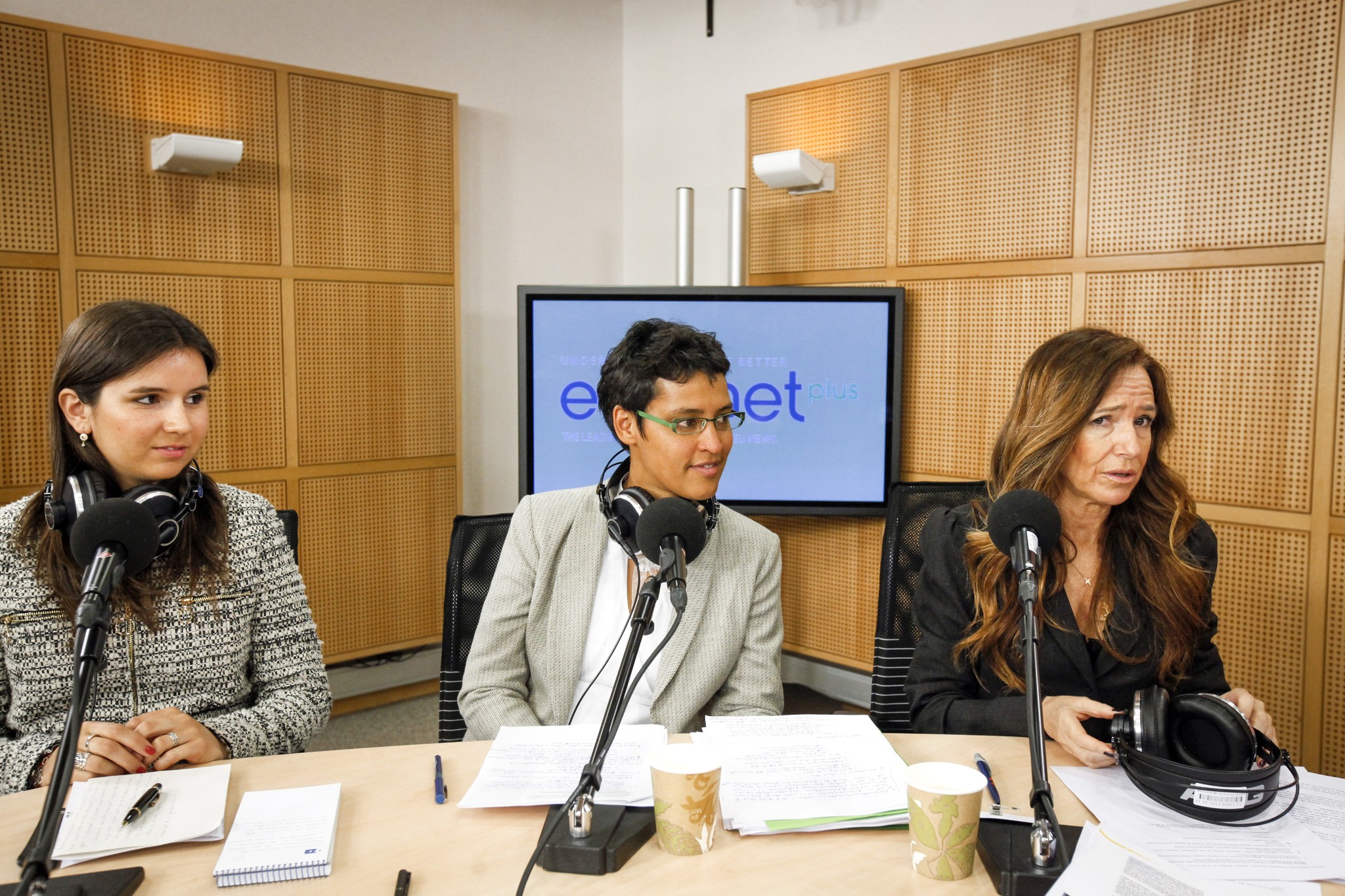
top-left (213, 784), bottom-right (342, 889)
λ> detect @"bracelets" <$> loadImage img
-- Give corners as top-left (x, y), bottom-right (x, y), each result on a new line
top-left (33, 743), bottom-right (59, 787)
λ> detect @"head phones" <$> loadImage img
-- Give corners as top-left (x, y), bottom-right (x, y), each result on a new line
top-left (43, 458), bottom-right (203, 559)
top-left (1104, 685), bottom-right (1301, 827)
top-left (596, 450), bottom-right (719, 558)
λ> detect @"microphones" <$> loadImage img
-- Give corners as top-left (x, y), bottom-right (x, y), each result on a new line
top-left (637, 496), bottom-right (706, 613)
top-left (987, 489), bottom-right (1061, 601)
top-left (70, 498), bottom-right (160, 629)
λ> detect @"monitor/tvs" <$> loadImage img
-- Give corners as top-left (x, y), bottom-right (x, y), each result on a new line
top-left (516, 285), bottom-right (900, 523)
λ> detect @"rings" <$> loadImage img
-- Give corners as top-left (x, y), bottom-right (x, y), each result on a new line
top-left (85, 734), bottom-right (101, 753)
top-left (74, 752), bottom-right (92, 771)
top-left (167, 732), bottom-right (179, 748)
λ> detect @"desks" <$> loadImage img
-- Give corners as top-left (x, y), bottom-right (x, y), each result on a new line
top-left (0, 734), bottom-right (1345, 896)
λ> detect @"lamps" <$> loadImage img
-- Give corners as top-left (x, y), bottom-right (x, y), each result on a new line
top-left (752, 148), bottom-right (835, 195)
top-left (151, 133), bottom-right (244, 175)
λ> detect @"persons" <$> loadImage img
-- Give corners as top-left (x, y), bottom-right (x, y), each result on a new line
top-left (0, 299), bottom-right (333, 796)
top-left (903, 327), bottom-right (1279, 770)
top-left (456, 318), bottom-right (784, 743)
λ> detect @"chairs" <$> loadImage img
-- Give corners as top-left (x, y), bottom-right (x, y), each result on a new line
top-left (438, 513), bottom-right (512, 743)
top-left (870, 480), bottom-right (990, 734)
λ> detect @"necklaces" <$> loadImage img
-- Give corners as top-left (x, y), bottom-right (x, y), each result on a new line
top-left (1067, 557), bottom-right (1111, 621)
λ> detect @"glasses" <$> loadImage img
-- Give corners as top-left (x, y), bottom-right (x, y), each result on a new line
top-left (633, 409), bottom-right (747, 436)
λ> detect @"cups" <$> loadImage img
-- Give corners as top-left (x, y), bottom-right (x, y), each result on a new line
top-left (904, 762), bottom-right (988, 881)
top-left (648, 742), bottom-right (724, 857)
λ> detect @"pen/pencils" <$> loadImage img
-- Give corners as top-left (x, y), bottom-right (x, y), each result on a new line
top-left (974, 752), bottom-right (1001, 805)
top-left (394, 868), bottom-right (411, 896)
top-left (121, 783), bottom-right (163, 829)
top-left (436, 754), bottom-right (449, 803)
